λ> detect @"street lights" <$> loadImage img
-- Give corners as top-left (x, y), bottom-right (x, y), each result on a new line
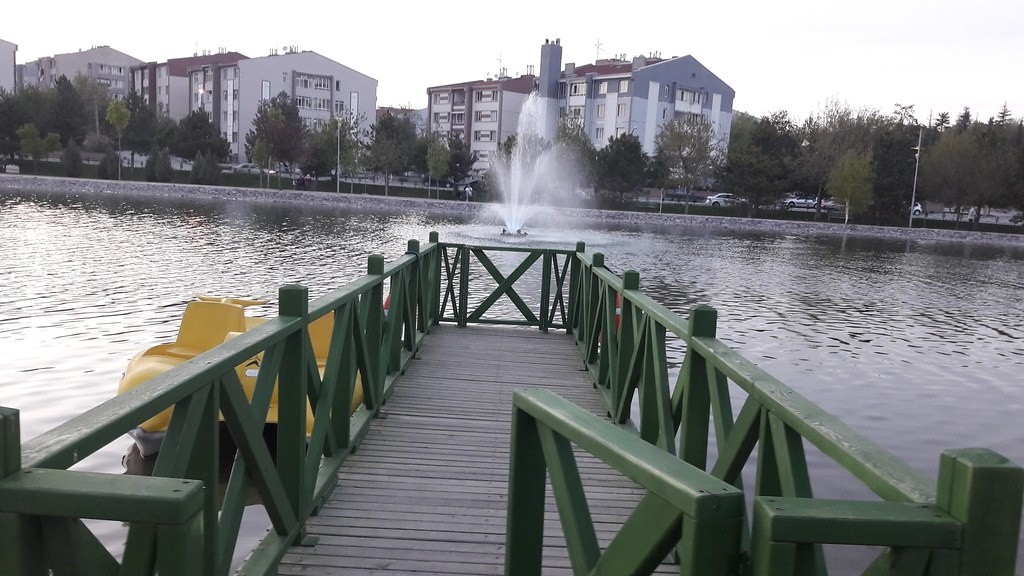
top-left (333, 115), bottom-right (342, 194)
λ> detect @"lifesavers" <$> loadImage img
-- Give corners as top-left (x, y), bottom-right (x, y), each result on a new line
top-left (383, 292), bottom-right (391, 320)
top-left (615, 291), bottom-right (622, 330)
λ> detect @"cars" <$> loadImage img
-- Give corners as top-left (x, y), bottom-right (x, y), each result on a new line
top-left (782, 196), bottom-right (817, 209)
top-left (705, 193), bottom-right (746, 208)
top-left (663, 187), bottom-right (705, 204)
top-left (422, 174), bottom-right (455, 187)
top-left (464, 180), bottom-right (487, 193)
top-left (813, 197), bottom-right (845, 210)
top-left (908, 202), bottom-right (922, 216)
top-left (235, 163), bottom-right (277, 175)
